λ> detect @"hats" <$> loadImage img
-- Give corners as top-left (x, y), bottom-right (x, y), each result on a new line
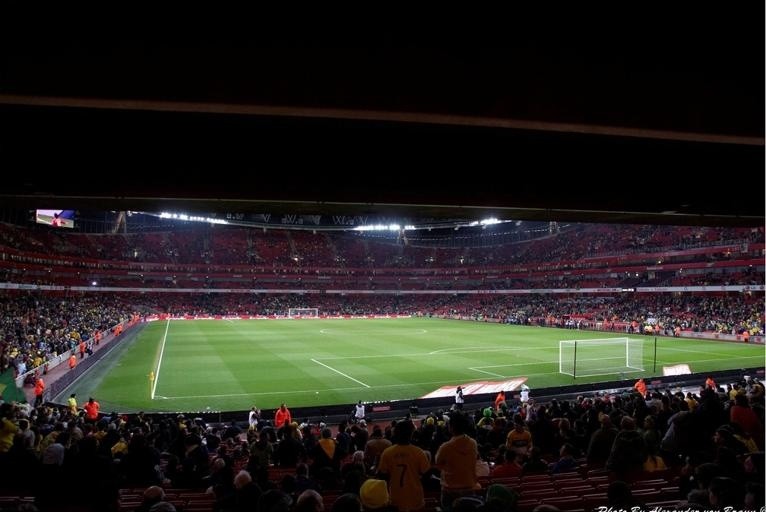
top-left (4, 406), bottom-right (495, 507)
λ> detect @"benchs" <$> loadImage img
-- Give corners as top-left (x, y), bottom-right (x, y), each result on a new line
top-left (117, 484), bottom-right (219, 511)
top-left (476, 469), bottom-right (691, 512)
